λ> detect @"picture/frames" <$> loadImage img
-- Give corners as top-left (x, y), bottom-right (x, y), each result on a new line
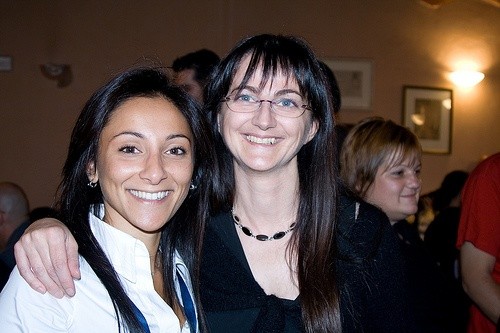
top-left (401, 84), bottom-right (454, 155)
top-left (316, 55), bottom-right (376, 112)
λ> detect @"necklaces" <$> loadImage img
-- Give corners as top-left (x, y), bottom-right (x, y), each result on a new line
top-left (233, 211), bottom-right (297, 241)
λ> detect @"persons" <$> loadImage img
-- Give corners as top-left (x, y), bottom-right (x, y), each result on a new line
top-left (0, 66), bottom-right (213, 333)
top-left (319, 55), bottom-right (500, 333)
top-left (0, 183), bottom-right (30, 291)
top-left (11, 31), bottom-right (407, 333)
top-left (171, 48), bottom-right (222, 109)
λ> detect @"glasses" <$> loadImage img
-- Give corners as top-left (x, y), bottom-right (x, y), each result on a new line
top-left (217, 91), bottom-right (315, 119)
